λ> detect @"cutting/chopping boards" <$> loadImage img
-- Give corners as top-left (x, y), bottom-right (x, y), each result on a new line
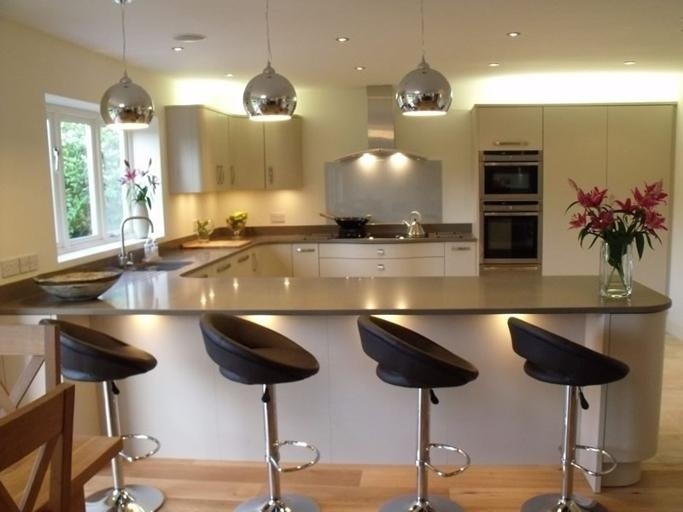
top-left (183, 239), bottom-right (251, 248)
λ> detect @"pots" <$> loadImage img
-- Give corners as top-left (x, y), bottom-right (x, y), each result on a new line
top-left (318, 212), bottom-right (369, 229)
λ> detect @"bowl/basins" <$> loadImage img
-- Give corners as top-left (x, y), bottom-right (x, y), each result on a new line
top-left (32, 266), bottom-right (124, 304)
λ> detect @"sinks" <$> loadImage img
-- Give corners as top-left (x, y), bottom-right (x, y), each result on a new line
top-left (136, 261), bottom-right (194, 272)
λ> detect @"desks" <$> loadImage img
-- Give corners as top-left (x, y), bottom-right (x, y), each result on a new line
top-left (0, 433), bottom-right (124, 512)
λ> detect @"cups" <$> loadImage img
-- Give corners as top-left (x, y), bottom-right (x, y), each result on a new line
top-left (193, 217), bottom-right (214, 242)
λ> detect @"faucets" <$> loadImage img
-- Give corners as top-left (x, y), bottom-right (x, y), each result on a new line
top-left (120, 215), bottom-right (154, 256)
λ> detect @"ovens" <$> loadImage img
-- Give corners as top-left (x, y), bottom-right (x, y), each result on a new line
top-left (478, 150), bottom-right (544, 276)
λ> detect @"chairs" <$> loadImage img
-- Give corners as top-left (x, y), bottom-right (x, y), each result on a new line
top-left (0, 380), bottom-right (74, 512)
top-left (196, 309), bottom-right (323, 512)
top-left (507, 315), bottom-right (630, 512)
top-left (0, 321), bottom-right (60, 414)
top-left (355, 312), bottom-right (478, 512)
top-left (36, 318), bottom-right (167, 512)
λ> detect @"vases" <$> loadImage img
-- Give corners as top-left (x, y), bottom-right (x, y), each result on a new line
top-left (128, 197), bottom-right (150, 238)
top-left (598, 241), bottom-right (634, 300)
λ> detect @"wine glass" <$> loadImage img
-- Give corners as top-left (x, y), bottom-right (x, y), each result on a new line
top-left (225, 208), bottom-right (250, 241)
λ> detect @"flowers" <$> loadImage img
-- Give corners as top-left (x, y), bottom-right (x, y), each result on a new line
top-left (562, 171), bottom-right (671, 292)
top-left (112, 150), bottom-right (157, 210)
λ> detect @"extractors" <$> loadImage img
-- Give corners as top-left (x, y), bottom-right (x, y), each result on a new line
top-left (333, 84), bottom-right (427, 163)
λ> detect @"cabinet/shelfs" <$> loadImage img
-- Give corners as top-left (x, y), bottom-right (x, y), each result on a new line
top-left (542, 102), bottom-right (678, 297)
top-left (468, 103), bottom-right (541, 153)
top-left (164, 104), bottom-right (305, 197)
top-left (181, 242), bottom-right (479, 278)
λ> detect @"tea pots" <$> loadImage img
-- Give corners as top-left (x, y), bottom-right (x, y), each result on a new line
top-left (402, 211), bottom-right (427, 239)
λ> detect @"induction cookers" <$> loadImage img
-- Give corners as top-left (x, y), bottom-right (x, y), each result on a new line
top-left (328, 232), bottom-right (431, 241)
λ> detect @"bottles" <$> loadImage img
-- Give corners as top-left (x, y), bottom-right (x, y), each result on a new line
top-left (143, 239), bottom-right (158, 260)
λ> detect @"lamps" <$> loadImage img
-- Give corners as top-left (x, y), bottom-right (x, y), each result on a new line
top-left (99, 0), bottom-right (154, 130)
top-left (241, 1), bottom-right (297, 122)
top-left (389, 0), bottom-right (454, 118)
